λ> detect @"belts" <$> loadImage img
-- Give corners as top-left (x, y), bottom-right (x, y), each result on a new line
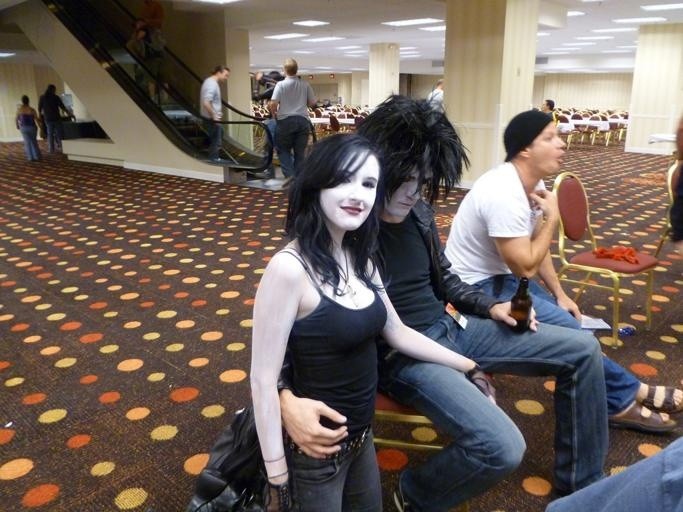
top-left (289, 428), bottom-right (368, 458)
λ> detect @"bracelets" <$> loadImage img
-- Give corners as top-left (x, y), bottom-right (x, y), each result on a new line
top-left (263, 455), bottom-right (289, 480)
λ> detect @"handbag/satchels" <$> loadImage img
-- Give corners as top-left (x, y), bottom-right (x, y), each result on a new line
top-left (188, 404), bottom-right (267, 512)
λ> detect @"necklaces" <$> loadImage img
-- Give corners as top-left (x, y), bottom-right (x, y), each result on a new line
top-left (333, 254), bottom-right (359, 299)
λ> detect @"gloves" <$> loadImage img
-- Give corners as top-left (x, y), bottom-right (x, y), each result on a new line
top-left (466, 366), bottom-right (497, 398)
top-left (266, 480), bottom-right (291, 511)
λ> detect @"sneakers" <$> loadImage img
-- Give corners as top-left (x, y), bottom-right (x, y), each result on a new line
top-left (392, 488), bottom-right (423, 511)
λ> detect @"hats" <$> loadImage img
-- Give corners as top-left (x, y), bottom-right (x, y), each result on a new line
top-left (504, 110), bottom-right (552, 162)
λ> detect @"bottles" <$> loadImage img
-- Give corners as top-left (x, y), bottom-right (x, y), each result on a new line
top-left (511, 276), bottom-right (533, 333)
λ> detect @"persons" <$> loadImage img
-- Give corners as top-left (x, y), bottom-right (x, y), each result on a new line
top-left (542, 435), bottom-right (683, 512)
top-left (245, 129), bottom-right (498, 512)
top-left (426, 78), bottom-right (445, 115)
top-left (274, 90), bottom-right (612, 511)
top-left (130, 0), bottom-right (166, 79)
top-left (250, 69), bottom-right (322, 181)
top-left (539, 98), bottom-right (554, 123)
top-left (37, 82), bottom-right (77, 155)
top-left (442, 109), bottom-right (682, 434)
top-left (15, 95), bottom-right (43, 162)
top-left (198, 63), bottom-right (232, 166)
top-left (266, 57), bottom-right (318, 188)
top-left (135, 17), bottom-right (172, 109)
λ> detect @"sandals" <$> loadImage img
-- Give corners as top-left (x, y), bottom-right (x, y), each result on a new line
top-left (609, 401), bottom-right (676, 433)
top-left (642, 386), bottom-right (683, 413)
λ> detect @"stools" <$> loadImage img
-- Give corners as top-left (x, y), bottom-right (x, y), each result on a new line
top-left (374, 371), bottom-right (496, 510)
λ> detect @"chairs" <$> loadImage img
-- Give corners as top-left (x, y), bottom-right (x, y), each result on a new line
top-left (651, 160), bottom-right (681, 258)
top-left (543, 107), bottom-right (629, 150)
top-left (252, 101), bottom-right (370, 146)
top-left (552, 172), bottom-right (658, 350)
